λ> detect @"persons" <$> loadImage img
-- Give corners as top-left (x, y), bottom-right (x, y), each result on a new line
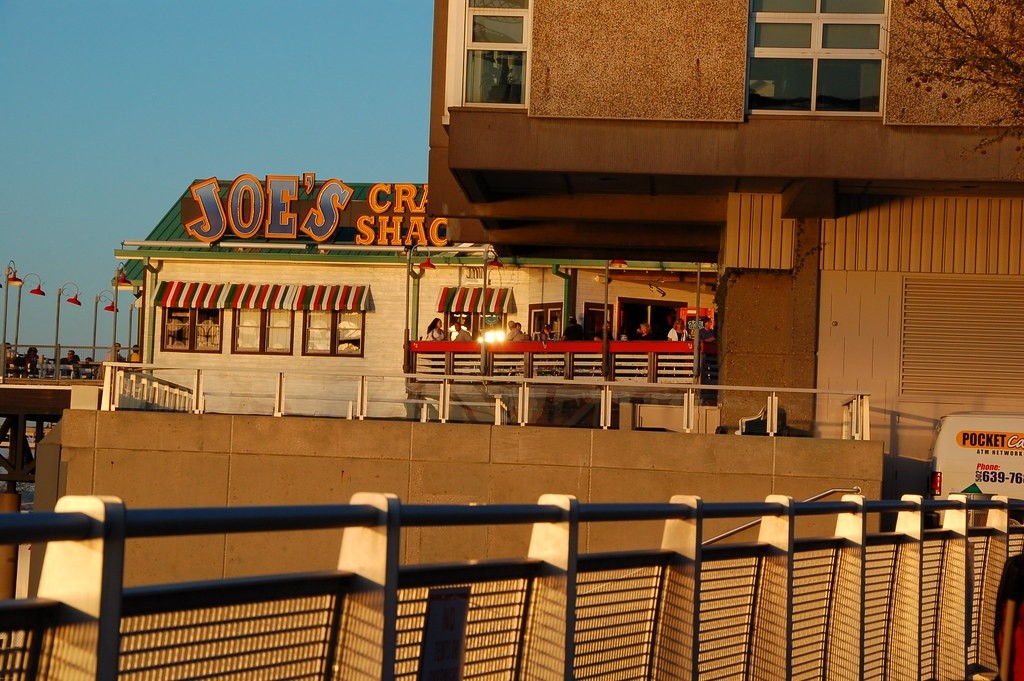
top-left (630, 323), bottom-right (652, 376)
top-left (665, 319), bottom-right (694, 377)
top-left (594, 322), bottom-right (614, 375)
top-left (106, 343), bottom-right (125, 361)
top-left (539, 326), bottom-right (551, 368)
top-left (426, 318), bottom-right (445, 383)
top-left (127, 345), bottom-right (140, 362)
top-left (60, 350), bottom-right (93, 379)
top-left (453, 322), bottom-right (472, 368)
top-left (503, 321), bottom-right (524, 384)
top-left (561, 319), bottom-right (585, 368)
top-left (5, 342), bottom-right (52, 378)
top-left (700, 318), bottom-right (716, 381)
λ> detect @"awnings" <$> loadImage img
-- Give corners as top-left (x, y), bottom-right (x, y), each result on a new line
top-left (135, 296), bottom-right (142, 307)
top-left (434, 287), bottom-right (517, 313)
top-left (151, 281), bottom-right (374, 312)
